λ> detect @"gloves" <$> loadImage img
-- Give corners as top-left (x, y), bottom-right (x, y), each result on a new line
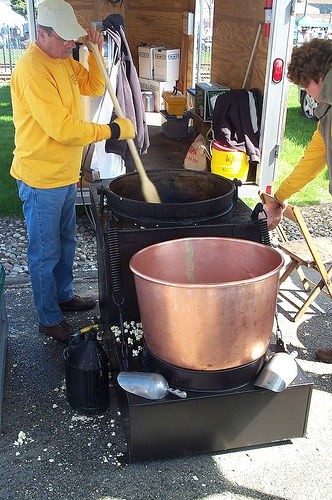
top-left (107, 117), bottom-right (135, 141)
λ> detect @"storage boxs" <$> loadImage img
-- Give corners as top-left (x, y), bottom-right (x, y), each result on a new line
top-left (136, 44), bottom-right (230, 141)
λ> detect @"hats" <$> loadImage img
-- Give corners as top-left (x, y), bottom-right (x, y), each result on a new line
top-left (37, 0), bottom-right (88, 40)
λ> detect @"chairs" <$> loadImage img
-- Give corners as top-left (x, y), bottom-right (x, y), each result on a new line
top-left (258, 190), bottom-right (332, 322)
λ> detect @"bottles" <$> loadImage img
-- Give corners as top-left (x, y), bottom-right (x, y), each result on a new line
top-left (142, 91), bottom-right (155, 112)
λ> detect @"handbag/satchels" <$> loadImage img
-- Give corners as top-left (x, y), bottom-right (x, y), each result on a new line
top-left (183, 134), bottom-right (212, 171)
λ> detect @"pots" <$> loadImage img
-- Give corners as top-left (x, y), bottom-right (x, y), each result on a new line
top-left (129, 236), bottom-right (285, 371)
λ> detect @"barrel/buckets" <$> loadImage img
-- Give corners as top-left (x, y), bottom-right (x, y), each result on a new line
top-left (63, 342), bottom-right (110, 416)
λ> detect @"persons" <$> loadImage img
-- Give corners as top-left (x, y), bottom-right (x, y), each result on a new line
top-left (259, 38), bottom-right (332, 364)
top-left (8, 0), bottom-right (137, 341)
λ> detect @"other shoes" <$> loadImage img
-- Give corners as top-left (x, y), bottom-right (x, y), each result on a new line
top-left (38, 319), bottom-right (82, 343)
top-left (57, 295), bottom-right (96, 310)
top-left (315, 346), bottom-right (332, 363)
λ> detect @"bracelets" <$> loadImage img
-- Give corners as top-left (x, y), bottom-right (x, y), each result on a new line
top-left (108, 123), bottom-right (120, 139)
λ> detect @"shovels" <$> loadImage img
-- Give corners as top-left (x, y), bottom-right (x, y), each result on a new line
top-left (254, 349), bottom-right (300, 395)
top-left (116, 371), bottom-right (187, 400)
top-left (86, 38), bottom-right (163, 205)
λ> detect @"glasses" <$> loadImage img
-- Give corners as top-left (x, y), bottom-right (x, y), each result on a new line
top-left (50, 31), bottom-right (74, 46)
top-left (297, 79), bottom-right (305, 91)
